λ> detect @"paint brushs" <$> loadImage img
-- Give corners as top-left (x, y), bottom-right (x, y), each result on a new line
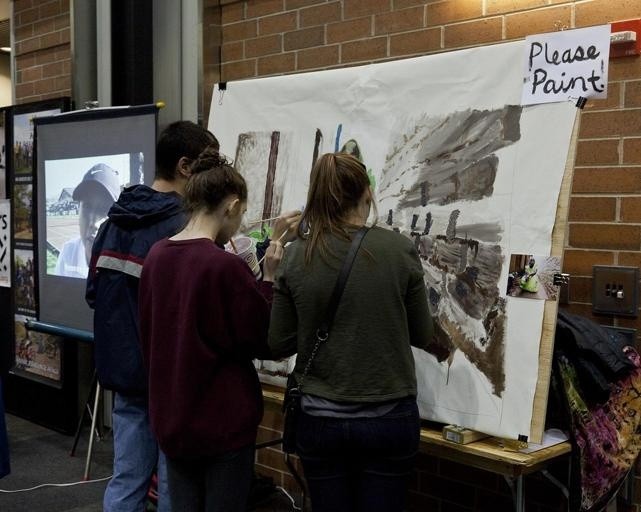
top-left (251, 225), bottom-right (290, 272)
top-left (245, 216), bottom-right (284, 226)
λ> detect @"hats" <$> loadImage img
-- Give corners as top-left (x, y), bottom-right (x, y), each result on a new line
top-left (72, 164), bottom-right (121, 202)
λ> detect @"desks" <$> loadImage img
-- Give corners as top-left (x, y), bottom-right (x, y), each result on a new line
top-left (259, 390), bottom-right (573, 512)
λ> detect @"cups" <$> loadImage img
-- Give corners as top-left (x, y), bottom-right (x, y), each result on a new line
top-left (228, 237), bottom-right (261, 280)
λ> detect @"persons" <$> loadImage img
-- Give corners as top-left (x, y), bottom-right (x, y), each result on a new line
top-left (513, 259), bottom-right (539, 293)
top-left (85, 121), bottom-right (305, 511)
top-left (136, 148), bottom-right (285, 512)
top-left (268, 153), bottom-right (434, 511)
top-left (55, 164), bottom-right (120, 279)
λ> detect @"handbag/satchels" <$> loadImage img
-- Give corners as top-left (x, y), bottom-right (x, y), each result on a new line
top-left (281, 374), bottom-right (300, 454)
